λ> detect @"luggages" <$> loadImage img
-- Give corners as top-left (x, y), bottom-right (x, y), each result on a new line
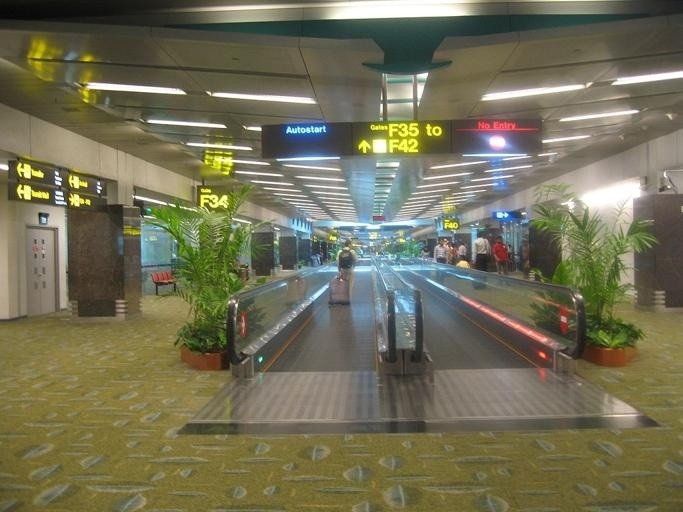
top-left (471, 261), bottom-right (486, 289)
top-left (328, 268), bottom-right (351, 305)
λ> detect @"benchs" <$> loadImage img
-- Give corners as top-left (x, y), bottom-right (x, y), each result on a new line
top-left (151, 272), bottom-right (177, 296)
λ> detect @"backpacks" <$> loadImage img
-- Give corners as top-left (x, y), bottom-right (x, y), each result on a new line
top-left (339, 248), bottom-right (354, 270)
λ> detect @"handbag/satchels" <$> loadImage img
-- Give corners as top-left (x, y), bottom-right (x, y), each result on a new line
top-left (486, 254), bottom-right (489, 262)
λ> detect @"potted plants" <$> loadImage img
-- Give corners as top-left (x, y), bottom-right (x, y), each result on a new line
top-left (142, 184), bottom-right (277, 369)
top-left (530, 183), bottom-right (661, 367)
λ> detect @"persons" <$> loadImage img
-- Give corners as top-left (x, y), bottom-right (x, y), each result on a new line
top-left (419, 231), bottom-right (513, 291)
top-left (335, 238), bottom-right (359, 303)
